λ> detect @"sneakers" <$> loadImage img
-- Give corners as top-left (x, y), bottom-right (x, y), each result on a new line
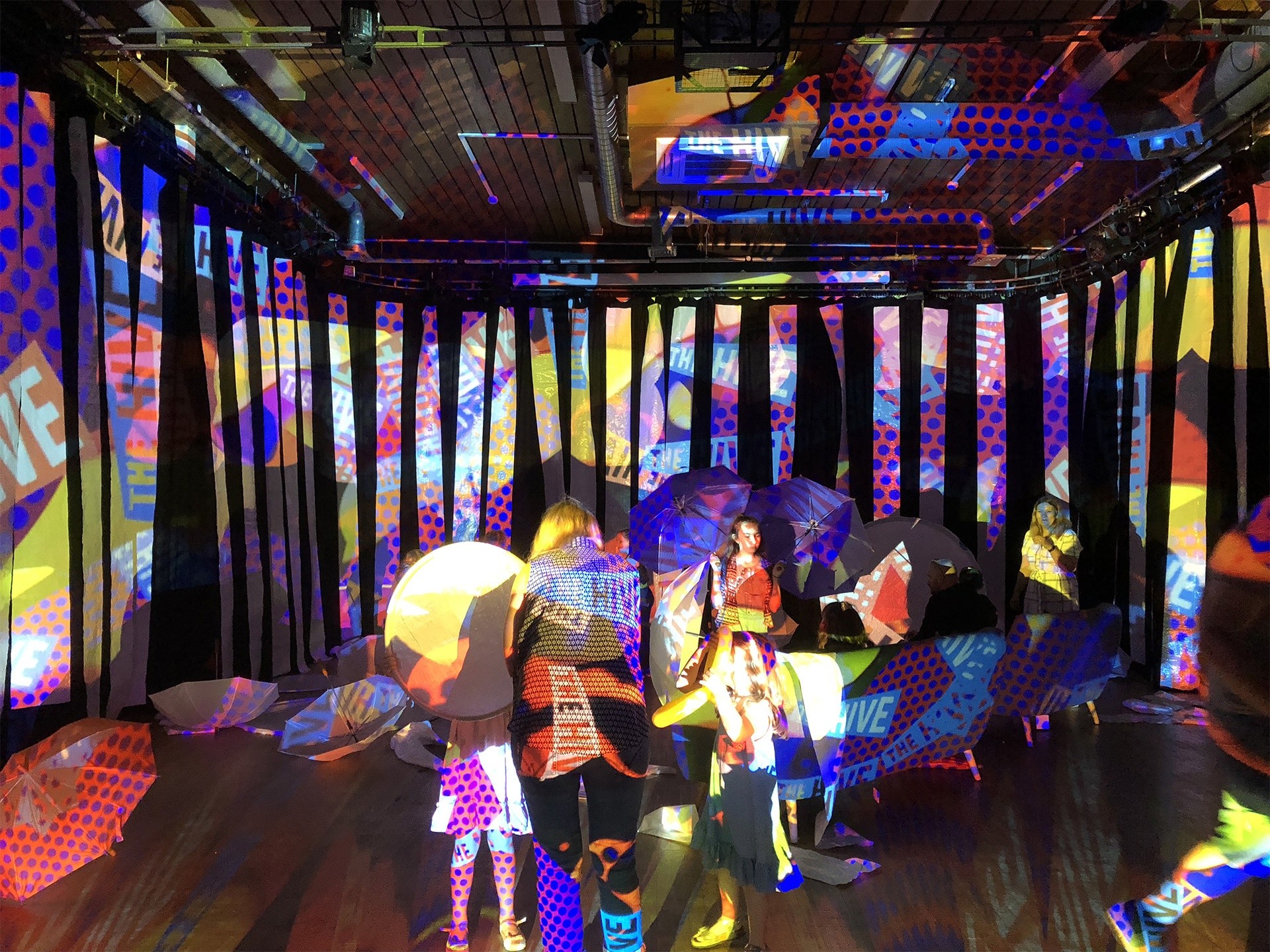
top-left (1103, 900), bottom-right (1164, 952)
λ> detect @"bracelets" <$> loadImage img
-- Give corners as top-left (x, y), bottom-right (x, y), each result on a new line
top-left (771, 573), bottom-right (780, 579)
top-left (1047, 545), bottom-right (1055, 553)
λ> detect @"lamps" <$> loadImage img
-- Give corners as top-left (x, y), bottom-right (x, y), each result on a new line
top-left (573, 0), bottom-right (649, 70)
top-left (279, 221), bottom-right (310, 251)
top-left (1150, 194), bottom-right (1191, 218)
top-left (1098, 0), bottom-right (1173, 52)
top-left (1115, 217), bottom-right (1149, 238)
top-left (309, 239), bottom-right (336, 269)
top-left (905, 279), bottom-right (926, 300)
top-left (336, 0), bottom-right (385, 74)
top-left (1083, 234), bottom-right (1123, 266)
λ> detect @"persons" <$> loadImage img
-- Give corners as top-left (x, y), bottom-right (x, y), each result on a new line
top-left (708, 515), bottom-right (787, 632)
top-left (1009, 496), bottom-right (1083, 615)
top-left (691, 630), bottom-right (786, 949)
top-left (818, 603), bottom-right (875, 652)
top-left (1106, 491), bottom-right (1270, 952)
top-left (502, 499), bottom-right (651, 952)
top-left (441, 709), bottom-right (527, 951)
top-left (615, 527), bottom-right (631, 554)
top-left (911, 558), bottom-right (999, 641)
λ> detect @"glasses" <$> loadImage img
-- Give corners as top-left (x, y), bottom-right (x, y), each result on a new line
top-left (931, 558), bottom-right (941, 576)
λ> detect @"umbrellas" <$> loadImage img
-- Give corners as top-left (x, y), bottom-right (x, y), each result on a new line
top-left (277, 657), bottom-right (407, 762)
top-left (337, 633), bottom-right (390, 680)
top-left (747, 472), bottom-right (874, 598)
top-left (1, 717), bottom-right (162, 903)
top-left (629, 464), bottom-right (753, 568)
top-left (149, 637), bottom-right (281, 736)
top-left (644, 553), bottom-right (731, 708)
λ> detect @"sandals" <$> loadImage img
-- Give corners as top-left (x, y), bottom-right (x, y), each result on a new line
top-left (440, 925), bottom-right (470, 952)
top-left (499, 917), bottom-right (526, 951)
top-left (690, 917), bottom-right (745, 949)
top-left (740, 939), bottom-right (771, 952)
